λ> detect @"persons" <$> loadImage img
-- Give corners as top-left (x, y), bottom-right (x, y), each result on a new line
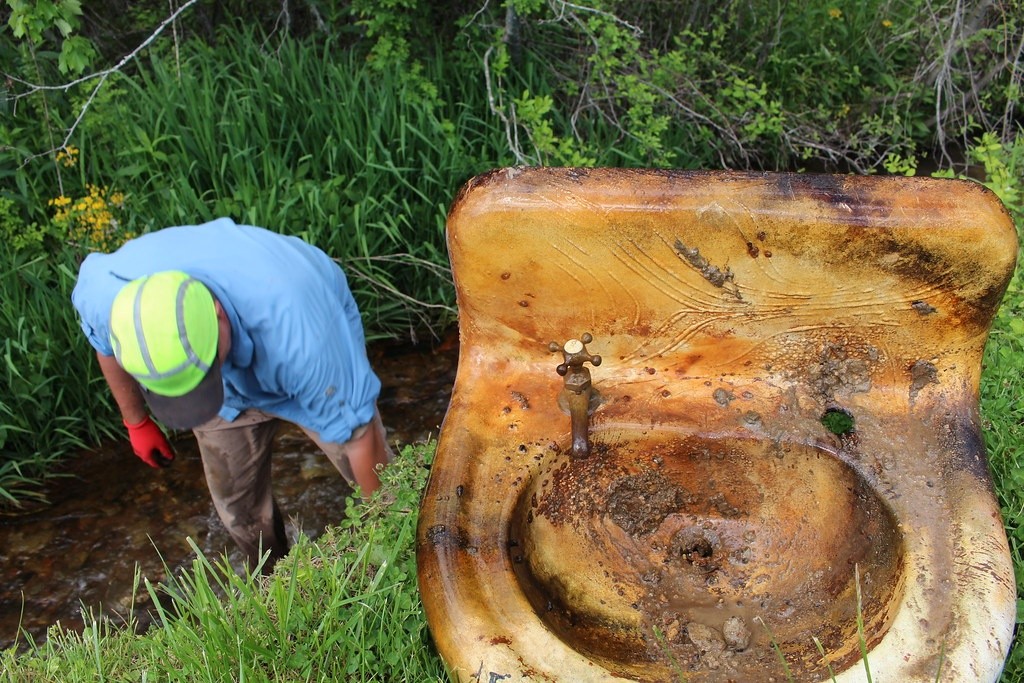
top-left (72, 217), bottom-right (396, 589)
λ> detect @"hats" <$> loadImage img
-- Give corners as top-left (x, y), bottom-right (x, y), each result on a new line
top-left (109, 269), bottom-right (224, 430)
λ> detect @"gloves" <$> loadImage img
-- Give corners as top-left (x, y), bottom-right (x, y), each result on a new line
top-left (123, 413), bottom-right (175, 470)
